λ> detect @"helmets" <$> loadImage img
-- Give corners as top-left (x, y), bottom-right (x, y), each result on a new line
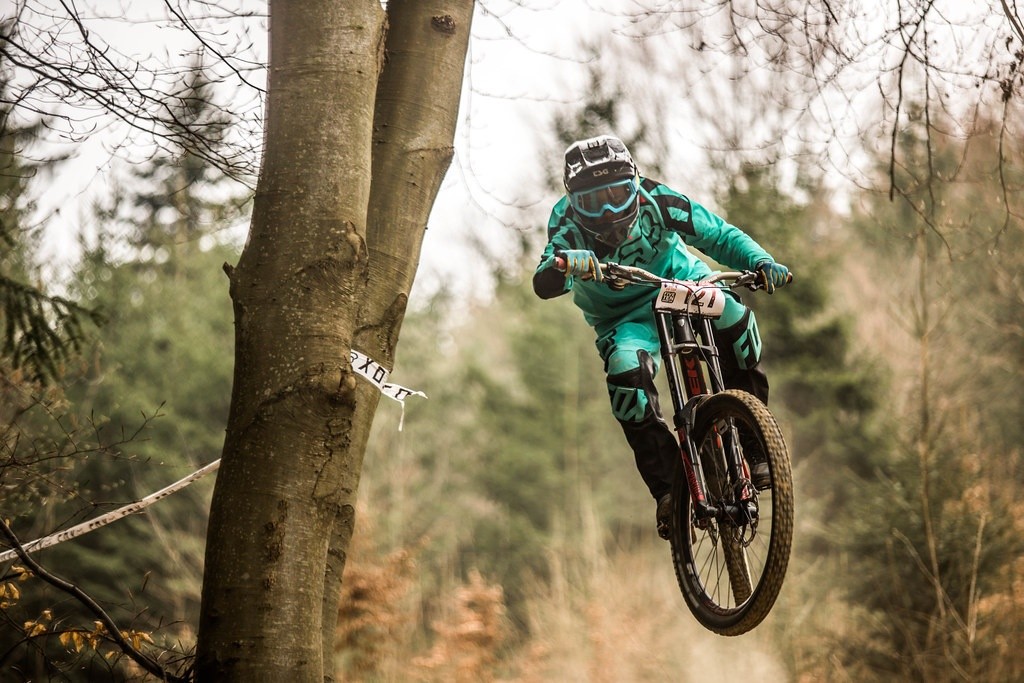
top-left (563, 132), bottom-right (641, 248)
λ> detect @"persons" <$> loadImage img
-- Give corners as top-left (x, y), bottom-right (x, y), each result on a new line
top-left (533, 134), bottom-right (794, 543)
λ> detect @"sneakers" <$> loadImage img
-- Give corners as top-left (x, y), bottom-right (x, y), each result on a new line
top-left (746, 453), bottom-right (775, 485)
top-left (652, 485), bottom-right (677, 539)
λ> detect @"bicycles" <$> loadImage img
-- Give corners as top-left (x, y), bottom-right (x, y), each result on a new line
top-left (553, 255), bottom-right (800, 637)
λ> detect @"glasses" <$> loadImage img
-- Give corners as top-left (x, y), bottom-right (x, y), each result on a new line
top-left (565, 164), bottom-right (645, 216)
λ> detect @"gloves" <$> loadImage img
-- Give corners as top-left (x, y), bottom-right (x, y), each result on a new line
top-left (756, 259), bottom-right (793, 296)
top-left (553, 245), bottom-right (605, 285)
